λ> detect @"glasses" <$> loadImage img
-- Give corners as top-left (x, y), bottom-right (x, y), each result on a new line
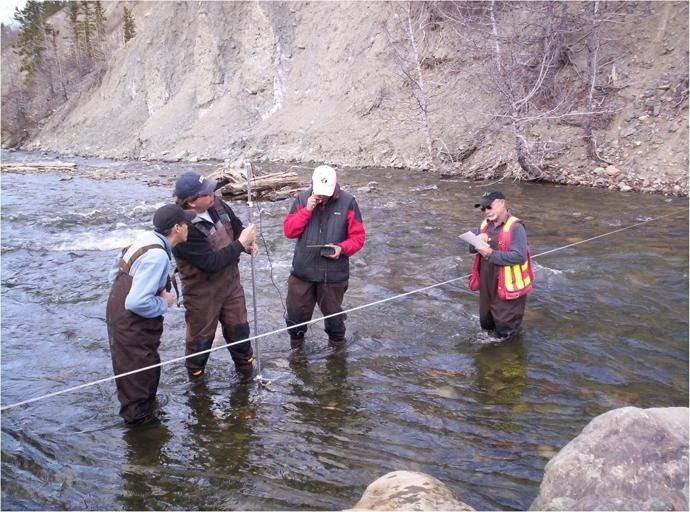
top-left (480, 205), bottom-right (492, 211)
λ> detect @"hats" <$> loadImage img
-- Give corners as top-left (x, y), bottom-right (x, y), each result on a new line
top-left (153, 204), bottom-right (196, 231)
top-left (474, 189), bottom-right (505, 209)
top-left (311, 164), bottom-right (338, 196)
top-left (175, 171), bottom-right (218, 199)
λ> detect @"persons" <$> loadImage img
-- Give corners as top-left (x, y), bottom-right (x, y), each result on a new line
top-left (106, 172), bottom-right (258, 423)
top-left (469, 190), bottom-right (534, 338)
top-left (284, 165), bottom-right (366, 350)
